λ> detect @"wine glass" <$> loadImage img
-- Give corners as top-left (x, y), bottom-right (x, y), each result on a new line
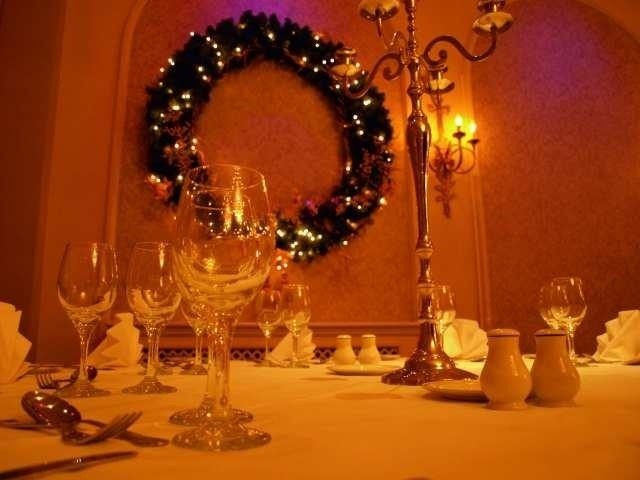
top-left (121, 241), bottom-right (183, 395)
top-left (171, 162), bottom-right (275, 453)
top-left (279, 284), bottom-right (312, 370)
top-left (57, 240), bottom-right (120, 399)
top-left (538, 278), bottom-right (591, 368)
top-left (255, 290), bottom-right (280, 367)
top-left (417, 284), bottom-right (457, 351)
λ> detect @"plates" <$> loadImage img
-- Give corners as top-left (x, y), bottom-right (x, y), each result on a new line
top-left (381, 354), bottom-right (400, 361)
top-left (423, 379), bottom-right (482, 402)
top-left (326, 364), bottom-right (401, 376)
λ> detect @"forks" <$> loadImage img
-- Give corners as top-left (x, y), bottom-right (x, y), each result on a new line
top-left (34, 368), bottom-right (63, 392)
top-left (4, 411), bottom-right (143, 446)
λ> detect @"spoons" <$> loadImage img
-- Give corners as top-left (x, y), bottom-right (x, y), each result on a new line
top-left (23, 392), bottom-right (168, 448)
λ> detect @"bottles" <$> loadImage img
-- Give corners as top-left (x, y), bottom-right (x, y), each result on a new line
top-left (479, 330), bottom-right (533, 412)
top-left (531, 329), bottom-right (580, 408)
top-left (357, 335), bottom-right (383, 363)
top-left (333, 334), bottom-right (356, 364)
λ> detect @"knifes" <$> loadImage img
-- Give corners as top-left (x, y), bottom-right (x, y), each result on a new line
top-left (1, 452), bottom-right (139, 480)
